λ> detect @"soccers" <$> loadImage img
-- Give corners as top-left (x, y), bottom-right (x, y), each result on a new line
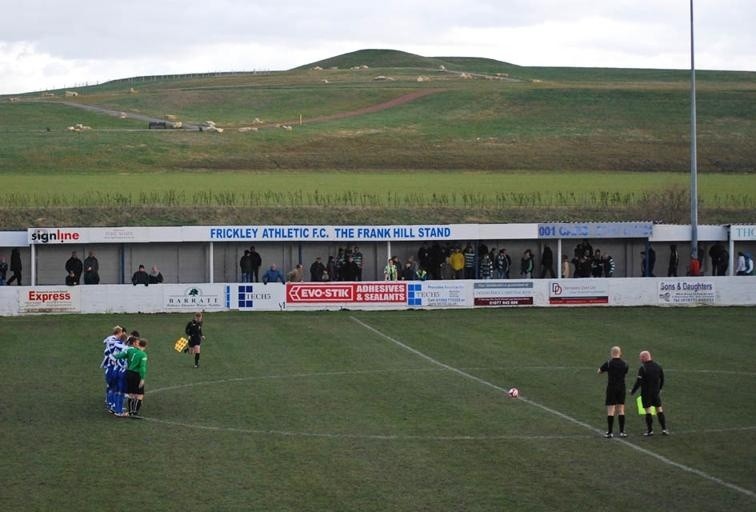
top-left (508, 388), bottom-right (519, 400)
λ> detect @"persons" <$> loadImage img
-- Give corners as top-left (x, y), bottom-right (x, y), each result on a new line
top-left (639, 242), bottom-right (678, 277)
top-left (384, 241), bottom-right (616, 282)
top-left (0, 256), bottom-right (8, 285)
top-left (630, 350), bottom-right (670, 435)
top-left (699, 242), bottom-right (755, 276)
top-left (597, 346), bottom-right (628, 436)
top-left (310, 247), bottom-right (363, 282)
top-left (150, 265), bottom-right (163, 283)
top-left (241, 247), bottom-right (262, 283)
top-left (184, 312), bottom-right (205, 369)
top-left (102, 326), bottom-right (148, 419)
top-left (131, 265), bottom-right (148, 286)
top-left (6, 248), bottom-right (21, 288)
top-left (65, 250), bottom-right (100, 287)
top-left (262, 264), bottom-right (304, 285)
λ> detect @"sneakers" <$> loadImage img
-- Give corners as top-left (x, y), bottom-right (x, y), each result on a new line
top-left (103, 400), bottom-right (145, 419)
top-left (603, 432), bottom-right (614, 438)
top-left (643, 431), bottom-right (654, 436)
top-left (662, 429), bottom-right (669, 436)
top-left (619, 431), bottom-right (629, 437)
top-left (192, 362), bottom-right (200, 368)
